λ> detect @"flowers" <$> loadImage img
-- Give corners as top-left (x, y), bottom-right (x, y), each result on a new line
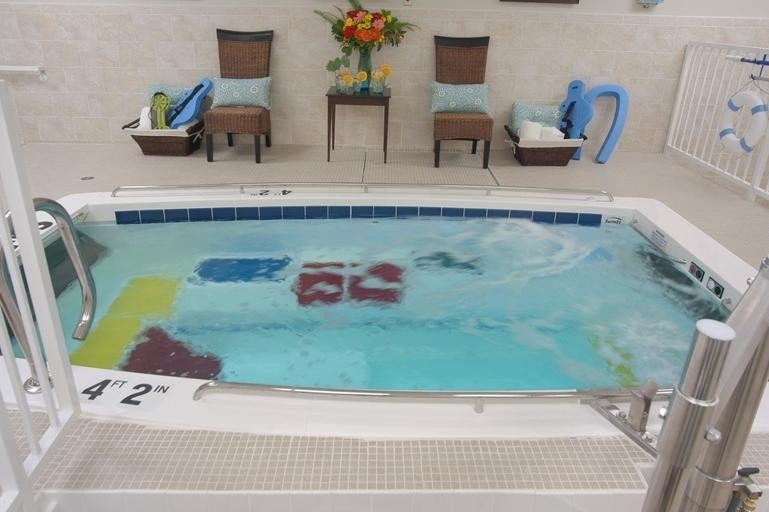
top-left (313, 0), bottom-right (422, 72)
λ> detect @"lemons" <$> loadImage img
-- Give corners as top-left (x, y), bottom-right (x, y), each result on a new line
top-left (381, 64), bottom-right (391, 70)
top-left (383, 67), bottom-right (390, 75)
top-left (343, 75), bottom-right (352, 86)
top-left (358, 71), bottom-right (367, 81)
top-left (353, 79), bottom-right (359, 83)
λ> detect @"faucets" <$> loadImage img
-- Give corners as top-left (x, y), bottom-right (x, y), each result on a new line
top-left (6, 197), bottom-right (97, 341)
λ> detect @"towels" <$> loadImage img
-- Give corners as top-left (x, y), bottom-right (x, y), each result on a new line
top-left (516, 121), bottom-right (564, 140)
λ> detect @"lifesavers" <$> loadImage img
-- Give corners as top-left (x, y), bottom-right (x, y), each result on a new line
top-left (719, 91), bottom-right (767, 154)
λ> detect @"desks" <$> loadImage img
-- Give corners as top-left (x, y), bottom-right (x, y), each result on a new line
top-left (325, 86), bottom-right (391, 163)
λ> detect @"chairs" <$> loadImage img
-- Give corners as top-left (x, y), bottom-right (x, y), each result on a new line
top-left (202, 28), bottom-right (274, 163)
top-left (433, 36), bottom-right (493, 168)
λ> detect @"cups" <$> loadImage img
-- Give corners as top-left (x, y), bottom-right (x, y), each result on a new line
top-left (335, 76), bottom-right (384, 95)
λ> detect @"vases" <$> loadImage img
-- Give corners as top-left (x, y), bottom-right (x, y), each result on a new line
top-left (358, 47), bottom-right (373, 89)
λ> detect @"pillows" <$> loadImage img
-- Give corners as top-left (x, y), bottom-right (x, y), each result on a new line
top-left (511, 102), bottom-right (560, 132)
top-left (432, 81), bottom-right (491, 115)
top-left (210, 76), bottom-right (272, 112)
